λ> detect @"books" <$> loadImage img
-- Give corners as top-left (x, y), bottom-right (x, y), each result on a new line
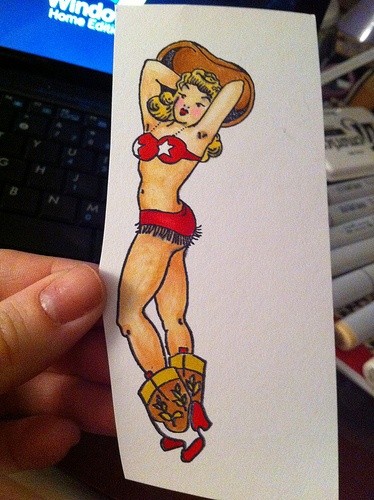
top-left (333, 295), bottom-right (374, 397)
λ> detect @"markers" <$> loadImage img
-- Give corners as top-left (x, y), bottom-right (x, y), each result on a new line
top-left (327, 175), bottom-right (374, 393)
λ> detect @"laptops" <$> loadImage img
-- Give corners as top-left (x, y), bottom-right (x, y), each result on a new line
top-left (0, 0), bottom-right (216, 265)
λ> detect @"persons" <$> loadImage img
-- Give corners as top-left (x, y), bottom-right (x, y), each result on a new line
top-left (1, 244), bottom-right (117, 476)
